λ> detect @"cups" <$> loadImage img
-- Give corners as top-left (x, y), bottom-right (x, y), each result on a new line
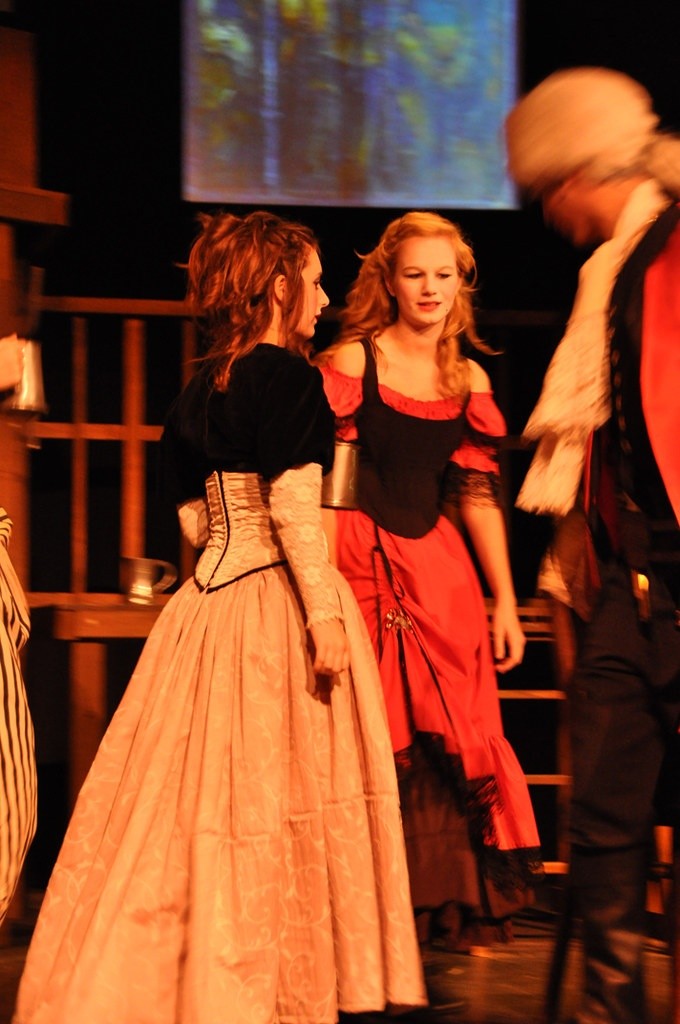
top-left (321, 442), bottom-right (360, 510)
top-left (120, 555), bottom-right (178, 606)
top-left (2, 339), bottom-right (47, 413)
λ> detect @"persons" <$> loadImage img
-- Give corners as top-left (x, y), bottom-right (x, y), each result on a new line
top-left (309, 211), bottom-right (541, 953)
top-left (11, 211), bottom-right (427, 1024)
top-left (503, 68), bottom-right (680, 1024)
top-left (0, 336), bottom-right (40, 929)
